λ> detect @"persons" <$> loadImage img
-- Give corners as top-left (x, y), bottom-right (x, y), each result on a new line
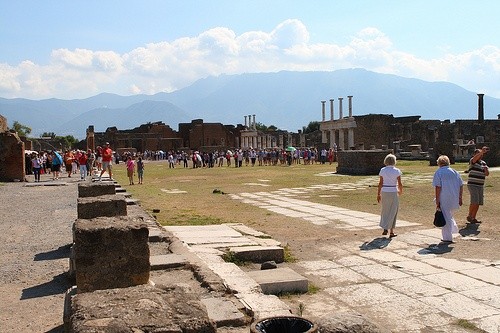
top-left (31, 153), bottom-right (42, 183)
top-left (466, 146), bottom-right (490, 224)
top-left (38, 145), bottom-right (337, 172)
top-left (136, 157), bottom-right (144, 185)
top-left (126, 156), bottom-right (136, 186)
top-left (64, 149), bottom-right (75, 178)
top-left (87, 149), bottom-right (96, 176)
top-left (78, 151), bottom-right (88, 181)
top-left (97, 142), bottom-right (116, 182)
top-left (377, 153), bottom-right (403, 237)
top-left (49, 149), bottom-right (63, 182)
top-left (432, 154), bottom-right (464, 243)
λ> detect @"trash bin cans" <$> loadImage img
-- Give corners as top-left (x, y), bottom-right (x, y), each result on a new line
top-left (429, 147), bottom-right (437, 166)
top-left (250, 315), bottom-right (319, 333)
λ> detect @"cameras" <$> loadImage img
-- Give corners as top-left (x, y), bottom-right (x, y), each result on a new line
top-left (484, 147), bottom-right (490, 151)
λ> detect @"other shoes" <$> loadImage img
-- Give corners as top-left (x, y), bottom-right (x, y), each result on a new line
top-left (390, 233), bottom-right (397, 237)
top-left (474, 218), bottom-right (482, 224)
top-left (382, 230), bottom-right (388, 235)
top-left (467, 216), bottom-right (475, 224)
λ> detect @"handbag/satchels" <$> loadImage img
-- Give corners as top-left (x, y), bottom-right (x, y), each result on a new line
top-left (433, 205), bottom-right (446, 227)
